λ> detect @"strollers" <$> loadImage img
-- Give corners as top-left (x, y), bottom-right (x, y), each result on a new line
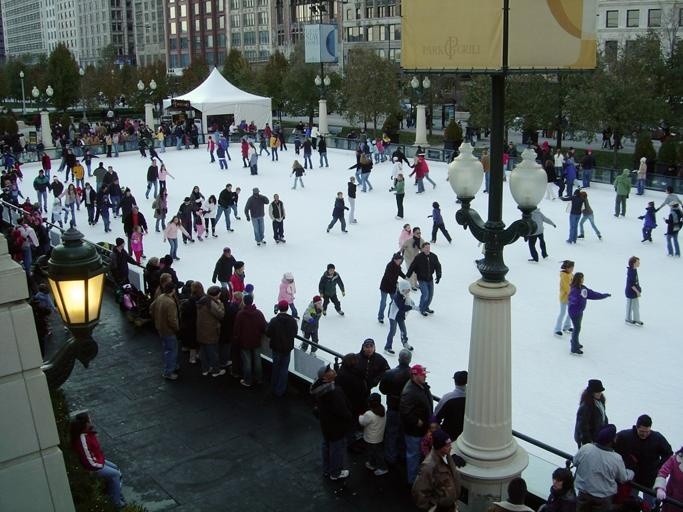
top-left (114, 283), bottom-right (150, 329)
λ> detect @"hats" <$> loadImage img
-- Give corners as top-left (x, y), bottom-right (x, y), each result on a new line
top-left (595, 423), bottom-right (618, 444)
top-left (410, 364), bottom-right (429, 376)
top-left (317, 362), bottom-right (333, 380)
top-left (588, 379), bottom-right (605, 394)
top-left (431, 428), bottom-right (450, 450)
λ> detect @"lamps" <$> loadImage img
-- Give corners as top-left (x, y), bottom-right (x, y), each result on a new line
top-left (41, 227), bottom-right (109, 395)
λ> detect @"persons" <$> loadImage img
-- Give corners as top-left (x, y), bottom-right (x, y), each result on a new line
top-left (2, 104), bottom-right (682, 511)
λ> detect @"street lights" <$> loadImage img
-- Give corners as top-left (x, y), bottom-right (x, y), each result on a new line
top-left (119, 23), bottom-right (152, 67)
top-left (411, 75), bottom-right (433, 149)
top-left (78, 67), bottom-right (86, 126)
top-left (30, 84), bottom-right (54, 149)
top-left (449, 127), bottom-right (550, 511)
top-left (308, 5), bottom-right (332, 138)
top-left (137, 79), bottom-right (157, 135)
top-left (19, 69), bottom-right (27, 115)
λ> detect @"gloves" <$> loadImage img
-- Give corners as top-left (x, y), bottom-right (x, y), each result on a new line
top-left (656, 487), bottom-right (668, 501)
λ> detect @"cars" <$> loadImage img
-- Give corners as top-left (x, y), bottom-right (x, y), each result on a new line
top-left (432, 103), bottom-right (470, 122)
top-left (400, 98), bottom-right (430, 117)
top-left (66, 101), bottom-right (86, 112)
top-left (624, 124), bottom-right (680, 140)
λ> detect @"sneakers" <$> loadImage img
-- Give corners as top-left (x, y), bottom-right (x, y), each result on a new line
top-left (342, 229), bottom-right (348, 232)
top-left (430, 240), bottom-right (437, 243)
top-left (352, 219), bottom-right (357, 223)
top-left (553, 327), bottom-right (584, 356)
top-left (365, 459), bottom-right (389, 476)
top-left (274, 304), bottom-right (278, 313)
top-left (162, 345), bottom-right (290, 397)
top-left (305, 164), bottom-right (328, 169)
top-left (526, 184), bottom-right (680, 264)
top-left (280, 237), bottom-right (286, 242)
top-left (422, 310), bottom-right (427, 315)
top-left (362, 189), bottom-right (366, 192)
top-left (211, 158), bottom-right (231, 169)
top-left (155, 216), bottom-right (241, 261)
top-left (64, 219), bottom-right (67, 224)
top-left (416, 189), bottom-right (425, 194)
top-left (322, 309), bottom-right (326, 316)
top-left (329, 469), bottom-right (350, 480)
top-left (275, 239), bottom-right (279, 243)
top-left (89, 214), bottom-right (121, 232)
top-left (327, 228), bottom-right (329, 232)
top-left (375, 157), bottom-right (392, 164)
top-left (626, 319), bottom-right (644, 326)
top-left (433, 183), bottom-right (436, 190)
top-left (141, 254), bottom-right (146, 258)
top-left (128, 252), bottom-right (132, 257)
top-left (257, 241), bottom-right (260, 245)
top-left (357, 183), bottom-right (362, 185)
top-left (411, 285), bottom-right (420, 293)
top-left (427, 308), bottom-right (434, 313)
top-left (89, 174), bottom-right (94, 177)
top-left (145, 194), bottom-right (149, 199)
top-left (378, 315), bottom-right (413, 355)
top-left (395, 216), bottom-right (403, 219)
top-left (261, 239), bottom-right (266, 243)
top-left (338, 310), bottom-right (344, 315)
top-left (368, 187), bottom-right (373, 192)
